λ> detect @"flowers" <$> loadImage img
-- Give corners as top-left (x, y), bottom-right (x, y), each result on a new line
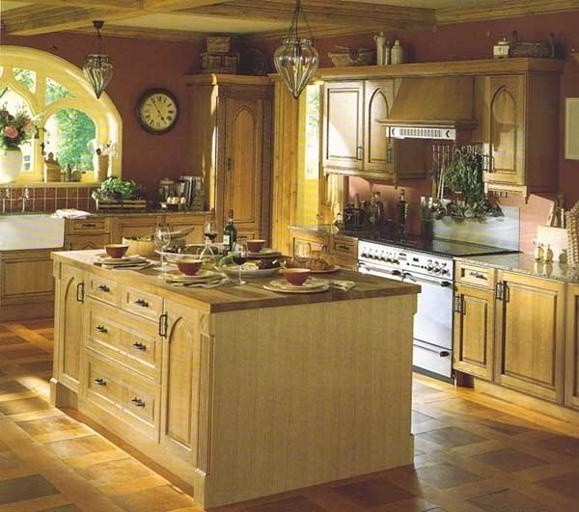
top-left (1, 104), bottom-right (49, 157)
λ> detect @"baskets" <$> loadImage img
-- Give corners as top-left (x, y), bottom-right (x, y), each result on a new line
top-left (120, 236), bottom-right (186, 257)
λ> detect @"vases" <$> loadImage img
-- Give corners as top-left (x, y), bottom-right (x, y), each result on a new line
top-left (2, 145), bottom-right (24, 182)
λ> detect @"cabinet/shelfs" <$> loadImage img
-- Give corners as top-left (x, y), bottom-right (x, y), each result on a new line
top-left (365, 65), bottom-right (429, 186)
top-left (288, 225), bottom-right (356, 270)
top-left (473, 57), bottom-right (563, 201)
top-left (81, 270), bottom-right (161, 470)
top-left (318, 66), bottom-right (365, 175)
top-left (162, 288), bottom-right (420, 510)
top-left (495, 269), bottom-right (565, 405)
top-left (183, 73), bottom-right (270, 245)
top-left (449, 258), bottom-right (494, 382)
top-left (565, 282), bottom-right (579, 411)
top-left (65, 209), bottom-right (110, 253)
top-left (104, 210), bottom-right (213, 248)
top-left (51, 257), bottom-right (81, 415)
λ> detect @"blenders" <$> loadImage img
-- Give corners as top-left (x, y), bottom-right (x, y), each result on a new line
top-left (158, 176), bottom-right (175, 210)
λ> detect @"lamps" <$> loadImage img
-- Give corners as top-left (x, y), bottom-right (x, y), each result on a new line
top-left (274, 1), bottom-right (320, 102)
top-left (80, 20), bottom-right (116, 99)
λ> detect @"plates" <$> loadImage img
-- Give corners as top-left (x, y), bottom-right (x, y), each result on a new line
top-left (90, 208), bottom-right (342, 295)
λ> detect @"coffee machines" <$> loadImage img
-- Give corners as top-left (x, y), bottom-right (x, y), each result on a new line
top-left (175, 175), bottom-right (204, 211)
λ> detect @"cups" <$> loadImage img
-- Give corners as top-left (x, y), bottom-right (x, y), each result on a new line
top-left (92, 154), bottom-right (110, 184)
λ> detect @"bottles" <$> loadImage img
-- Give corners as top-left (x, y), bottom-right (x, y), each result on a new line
top-left (371, 31), bottom-right (405, 66)
top-left (351, 187), bottom-right (410, 238)
top-left (71, 164), bottom-right (82, 182)
top-left (492, 37), bottom-right (513, 58)
top-left (534, 241), bottom-right (543, 262)
top-left (542, 261), bottom-right (553, 278)
top-left (535, 261), bottom-right (543, 274)
top-left (542, 244), bottom-right (553, 262)
top-left (63, 163), bottom-right (71, 182)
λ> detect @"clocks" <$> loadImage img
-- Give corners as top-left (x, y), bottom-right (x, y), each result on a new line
top-left (136, 88), bottom-right (177, 135)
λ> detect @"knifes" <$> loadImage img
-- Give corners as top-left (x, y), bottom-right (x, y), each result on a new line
top-left (545, 191), bottom-right (567, 228)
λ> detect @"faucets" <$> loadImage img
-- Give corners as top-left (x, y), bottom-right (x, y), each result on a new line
top-left (1, 184), bottom-right (11, 215)
top-left (21, 185), bottom-right (30, 213)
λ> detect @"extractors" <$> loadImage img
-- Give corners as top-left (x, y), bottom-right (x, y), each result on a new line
top-left (374, 75), bottom-right (477, 144)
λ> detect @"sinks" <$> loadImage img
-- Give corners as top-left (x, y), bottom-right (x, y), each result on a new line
top-left (0, 211), bottom-right (65, 251)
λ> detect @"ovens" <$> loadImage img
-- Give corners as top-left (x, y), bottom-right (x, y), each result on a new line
top-left (355, 240), bottom-right (455, 383)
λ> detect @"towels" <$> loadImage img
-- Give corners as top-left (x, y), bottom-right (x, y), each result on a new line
top-left (54, 206), bottom-right (91, 222)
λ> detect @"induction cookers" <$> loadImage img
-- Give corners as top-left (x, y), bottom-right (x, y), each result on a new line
top-left (358, 230), bottom-right (510, 256)
